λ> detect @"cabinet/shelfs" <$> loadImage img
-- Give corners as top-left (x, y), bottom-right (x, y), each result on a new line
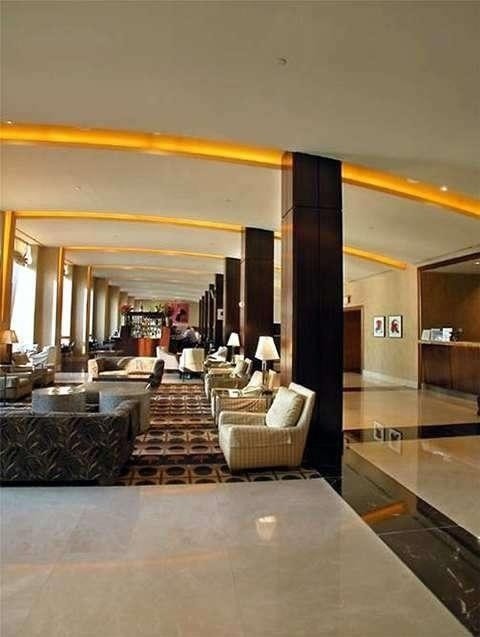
top-left (128, 311), bottom-right (164, 340)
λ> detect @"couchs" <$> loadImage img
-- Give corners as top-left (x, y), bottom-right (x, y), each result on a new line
top-left (0, 355), bottom-right (165, 482)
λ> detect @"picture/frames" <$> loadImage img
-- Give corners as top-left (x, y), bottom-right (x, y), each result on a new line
top-left (372, 420), bottom-right (403, 455)
top-left (374, 315), bottom-right (402, 337)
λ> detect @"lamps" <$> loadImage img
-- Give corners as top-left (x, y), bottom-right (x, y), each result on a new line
top-left (0, 330), bottom-right (19, 362)
top-left (226, 332), bottom-right (240, 361)
top-left (254, 336), bottom-right (280, 383)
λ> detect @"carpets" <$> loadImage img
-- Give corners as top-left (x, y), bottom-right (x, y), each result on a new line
top-left (119, 381), bottom-right (321, 485)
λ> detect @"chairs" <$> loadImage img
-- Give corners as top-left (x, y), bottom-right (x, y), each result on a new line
top-left (205, 354), bottom-right (315, 472)
top-left (156, 346), bottom-right (179, 372)
top-left (179, 347), bottom-right (205, 383)
